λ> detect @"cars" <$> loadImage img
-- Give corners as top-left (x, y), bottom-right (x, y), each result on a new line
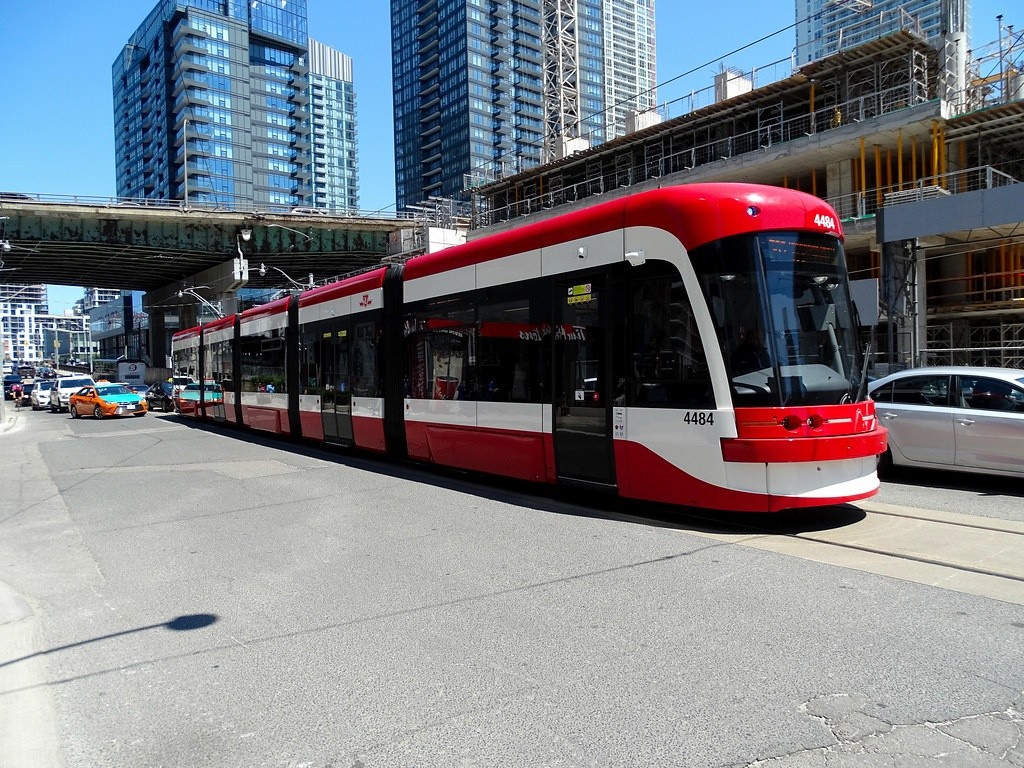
top-left (867, 365), bottom-right (1024, 478)
top-left (144, 377), bottom-right (174, 413)
top-left (49, 376), bottom-right (96, 412)
top-left (68, 379), bottom-right (149, 419)
top-left (0, 361), bottom-right (85, 406)
top-left (127, 384), bottom-right (150, 399)
top-left (30, 381), bottom-right (52, 411)
top-left (174, 352), bottom-right (604, 415)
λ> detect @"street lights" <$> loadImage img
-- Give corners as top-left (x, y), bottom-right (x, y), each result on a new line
top-left (183, 118), bottom-right (191, 208)
top-left (259, 263), bottom-right (314, 290)
top-left (107, 320), bottom-right (141, 362)
top-left (34, 324), bottom-right (59, 370)
top-left (71, 322), bottom-right (93, 375)
top-left (177, 288), bottom-right (223, 322)
top-left (0, 239), bottom-right (11, 251)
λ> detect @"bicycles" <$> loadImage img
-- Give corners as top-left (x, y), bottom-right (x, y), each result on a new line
top-left (13, 397), bottom-right (22, 411)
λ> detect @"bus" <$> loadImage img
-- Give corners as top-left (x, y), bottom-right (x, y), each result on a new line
top-left (173, 181), bottom-right (886, 513)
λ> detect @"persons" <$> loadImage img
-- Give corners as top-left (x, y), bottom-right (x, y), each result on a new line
top-left (12, 383), bottom-right (24, 406)
top-left (988, 384), bottom-right (1024, 411)
top-left (732, 323), bottom-right (771, 377)
top-left (257, 381), bottom-right (274, 403)
